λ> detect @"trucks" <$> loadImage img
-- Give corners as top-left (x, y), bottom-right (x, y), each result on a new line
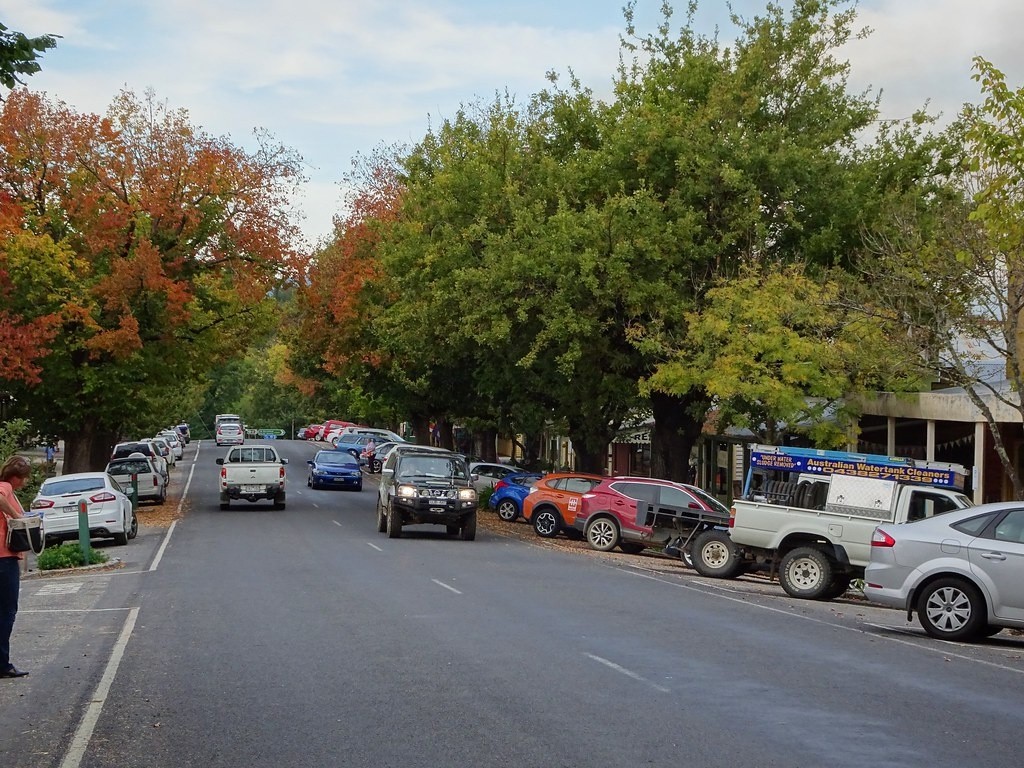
top-left (216, 414), bottom-right (243, 432)
top-left (110, 440), bottom-right (170, 486)
top-left (636, 500), bottom-right (780, 579)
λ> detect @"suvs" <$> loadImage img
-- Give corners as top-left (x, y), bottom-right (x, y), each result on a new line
top-left (376, 447), bottom-right (479, 541)
top-left (522, 471), bottom-right (606, 540)
top-left (574, 475), bottom-right (731, 553)
top-left (319, 420), bottom-right (357, 442)
top-left (338, 427), bottom-right (406, 441)
top-left (334, 435), bottom-right (392, 460)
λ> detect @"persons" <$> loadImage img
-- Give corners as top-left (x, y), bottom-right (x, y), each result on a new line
top-left (44, 445), bottom-right (57, 463)
top-left (363, 438), bottom-right (377, 474)
top-left (0, 456), bottom-right (32, 678)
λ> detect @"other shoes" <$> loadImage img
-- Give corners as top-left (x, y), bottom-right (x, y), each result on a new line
top-left (0, 667), bottom-right (30, 678)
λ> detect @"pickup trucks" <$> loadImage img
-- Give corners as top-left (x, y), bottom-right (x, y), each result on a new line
top-left (729, 477), bottom-right (1009, 600)
top-left (216, 445), bottom-right (288, 510)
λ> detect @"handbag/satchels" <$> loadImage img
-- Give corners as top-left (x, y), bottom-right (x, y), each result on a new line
top-left (1, 493), bottom-right (45, 556)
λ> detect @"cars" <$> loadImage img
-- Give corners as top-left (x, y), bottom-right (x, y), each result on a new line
top-left (488, 475), bottom-right (545, 522)
top-left (216, 425), bottom-right (244, 446)
top-left (861, 500), bottom-right (1024, 643)
top-left (382, 444), bottom-right (451, 479)
top-left (327, 428), bottom-right (343, 448)
top-left (296, 428), bottom-right (306, 440)
top-left (30, 472), bottom-right (138, 548)
top-left (157, 431), bottom-right (181, 442)
top-left (140, 437), bottom-right (176, 467)
top-left (307, 449), bottom-right (365, 492)
top-left (469, 462), bottom-right (530, 497)
top-left (104, 457), bottom-right (168, 504)
top-left (156, 434), bottom-right (183, 460)
top-left (358, 441), bottom-right (410, 473)
top-left (304, 424), bottom-right (324, 442)
top-left (167, 423), bottom-right (190, 447)
